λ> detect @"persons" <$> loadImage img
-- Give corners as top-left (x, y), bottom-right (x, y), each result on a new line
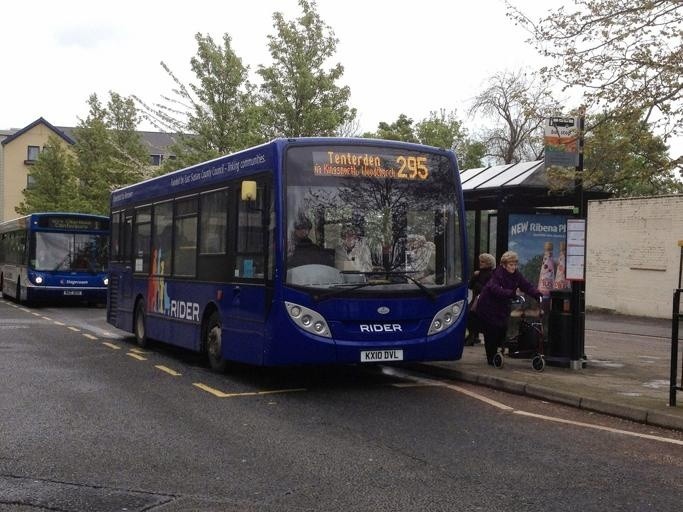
top-left (475, 250), bottom-right (542, 367)
top-left (333, 222), bottom-right (374, 270)
top-left (464, 252), bottom-right (496, 348)
top-left (287, 214), bottom-right (316, 269)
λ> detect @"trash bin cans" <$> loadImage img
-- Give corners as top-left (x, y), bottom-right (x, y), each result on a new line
top-left (546, 288), bottom-right (588, 368)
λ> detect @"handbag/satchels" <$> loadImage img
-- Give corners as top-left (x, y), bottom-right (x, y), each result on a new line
top-left (470, 295), bottom-right (480, 313)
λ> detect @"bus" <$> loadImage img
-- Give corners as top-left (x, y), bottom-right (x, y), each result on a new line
top-left (103, 133), bottom-right (475, 385)
top-left (0, 210), bottom-right (111, 309)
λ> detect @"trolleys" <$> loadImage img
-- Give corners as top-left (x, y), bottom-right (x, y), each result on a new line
top-left (489, 290), bottom-right (550, 374)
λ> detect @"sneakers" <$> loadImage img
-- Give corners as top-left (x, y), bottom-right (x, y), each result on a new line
top-left (487, 358), bottom-right (494, 365)
top-left (464, 336), bottom-right (481, 346)
